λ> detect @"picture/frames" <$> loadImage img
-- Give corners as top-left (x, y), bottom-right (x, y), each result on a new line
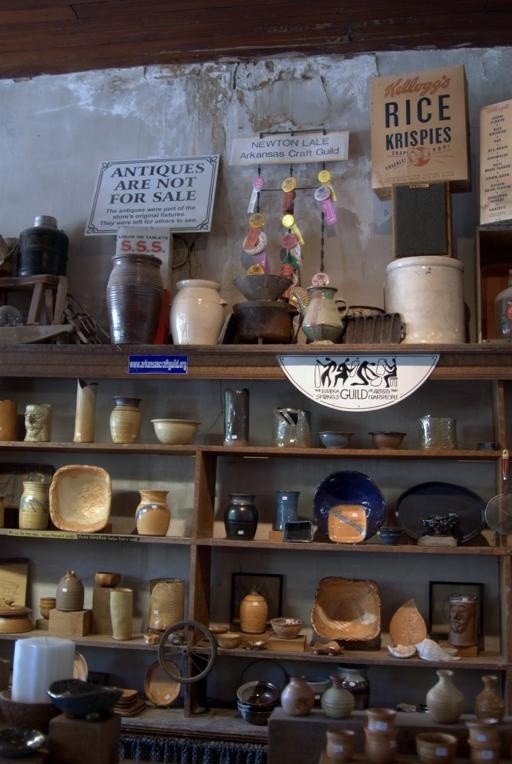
top-left (428, 581), bottom-right (486, 639)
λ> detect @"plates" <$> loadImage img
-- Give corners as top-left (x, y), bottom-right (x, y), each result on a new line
top-left (49, 463), bottom-right (112, 532)
top-left (395, 483), bottom-right (486, 543)
top-left (70, 651), bottom-right (90, 685)
top-left (142, 658), bottom-right (182, 707)
top-left (111, 687), bottom-right (144, 718)
top-left (387, 596), bottom-right (429, 652)
top-left (311, 471), bottom-right (388, 543)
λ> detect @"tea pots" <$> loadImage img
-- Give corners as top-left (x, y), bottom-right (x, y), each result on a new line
top-left (289, 284), bottom-right (349, 344)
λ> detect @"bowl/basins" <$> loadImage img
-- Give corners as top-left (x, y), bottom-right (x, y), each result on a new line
top-left (313, 572), bottom-right (383, 641)
top-left (235, 681), bottom-right (279, 725)
top-left (95, 570), bottom-right (120, 587)
top-left (1, 689), bottom-right (62, 734)
top-left (316, 430), bottom-right (353, 448)
top-left (207, 615), bottom-right (303, 649)
top-left (0, 724), bottom-right (46, 759)
top-left (151, 418), bottom-right (202, 446)
top-left (46, 675), bottom-right (122, 722)
top-left (367, 431), bottom-right (406, 448)
top-left (302, 673), bottom-right (330, 695)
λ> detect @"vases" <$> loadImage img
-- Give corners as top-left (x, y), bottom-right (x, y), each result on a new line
top-left (277, 663), bottom-right (507, 727)
top-left (103, 253), bottom-right (163, 344)
top-left (170, 280), bottom-right (227, 347)
top-left (0, 376), bottom-right (314, 650)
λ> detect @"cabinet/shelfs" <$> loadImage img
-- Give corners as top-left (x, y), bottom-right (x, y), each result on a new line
top-left (0, 346), bottom-right (512, 745)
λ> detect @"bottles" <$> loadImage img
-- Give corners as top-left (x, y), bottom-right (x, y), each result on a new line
top-left (282, 675), bottom-right (352, 718)
top-left (224, 493), bottom-right (258, 539)
top-left (276, 490), bottom-right (300, 530)
top-left (135, 489), bottom-right (170, 537)
top-left (384, 254), bottom-right (467, 343)
top-left (427, 670), bottom-right (500, 721)
top-left (19, 481), bottom-right (52, 528)
top-left (56, 569), bottom-right (84, 611)
top-left (106, 254), bottom-right (224, 346)
top-left (240, 590), bottom-right (269, 633)
top-left (110, 394), bottom-right (141, 445)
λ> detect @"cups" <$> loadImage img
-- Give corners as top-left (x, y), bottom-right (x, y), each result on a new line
top-left (417, 416), bottom-right (459, 450)
top-left (0, 398), bottom-right (54, 441)
top-left (39, 596), bottom-right (55, 620)
top-left (322, 707), bottom-right (501, 763)
top-left (109, 586), bottom-right (135, 641)
top-left (149, 578), bottom-right (185, 633)
top-left (275, 408), bottom-right (311, 447)
top-left (221, 387), bottom-right (250, 446)
